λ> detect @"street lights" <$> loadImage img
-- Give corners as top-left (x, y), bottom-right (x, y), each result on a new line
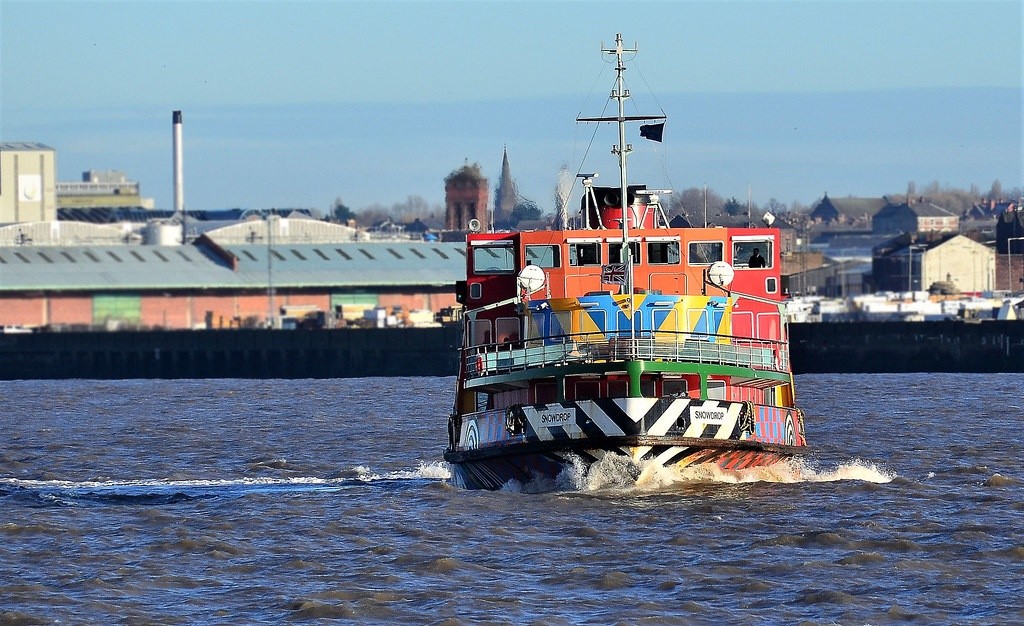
top-left (938, 240), bottom-right (960, 285)
top-left (908, 245), bottom-right (919, 293)
top-left (972, 240), bottom-right (998, 293)
top-left (1008, 236), bottom-right (1024, 291)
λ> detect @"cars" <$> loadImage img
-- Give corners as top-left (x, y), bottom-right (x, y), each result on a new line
top-left (3, 323), bottom-right (34, 334)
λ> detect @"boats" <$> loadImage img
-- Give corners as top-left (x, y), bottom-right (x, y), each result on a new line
top-left (442, 28), bottom-right (808, 499)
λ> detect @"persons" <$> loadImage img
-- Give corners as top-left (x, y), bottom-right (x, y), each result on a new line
top-left (749, 248), bottom-right (766, 268)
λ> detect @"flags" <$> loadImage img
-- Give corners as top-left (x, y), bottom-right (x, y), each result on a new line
top-left (640, 123), bottom-right (664, 142)
